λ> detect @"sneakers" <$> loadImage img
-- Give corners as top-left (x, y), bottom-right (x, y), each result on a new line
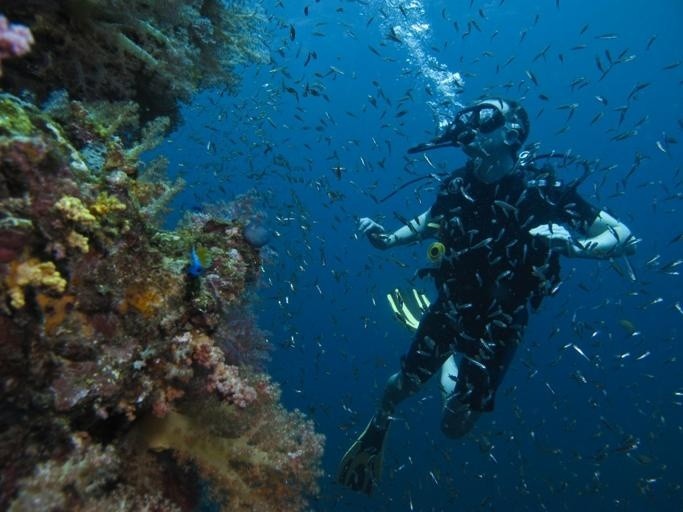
top-left (362, 411), bottom-right (393, 454)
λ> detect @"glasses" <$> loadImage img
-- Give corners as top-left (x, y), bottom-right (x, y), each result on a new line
top-left (456, 103), bottom-right (506, 133)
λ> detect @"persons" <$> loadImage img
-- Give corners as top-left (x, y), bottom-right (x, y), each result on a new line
top-left (336, 97), bottom-right (638, 497)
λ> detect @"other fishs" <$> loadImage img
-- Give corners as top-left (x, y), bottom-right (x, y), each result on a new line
top-left (138, 0), bottom-right (683, 511)
